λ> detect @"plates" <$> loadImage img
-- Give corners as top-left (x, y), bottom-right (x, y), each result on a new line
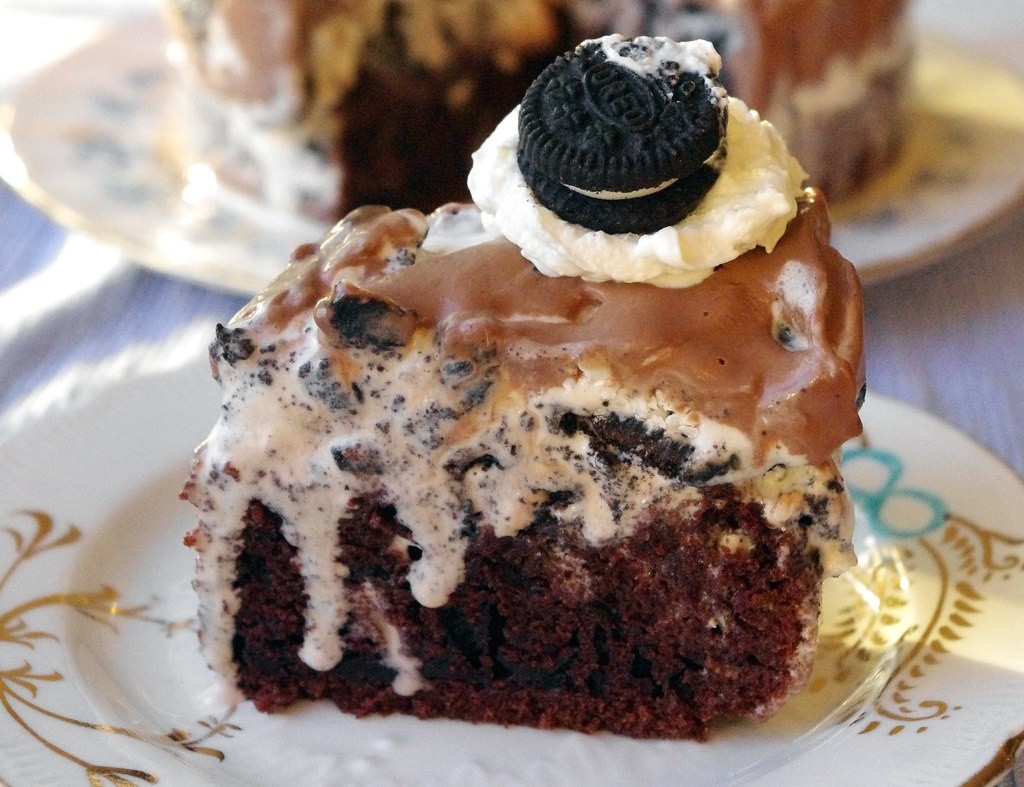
top-left (0, 315), bottom-right (1024, 787)
top-left (0, 27), bottom-right (1024, 299)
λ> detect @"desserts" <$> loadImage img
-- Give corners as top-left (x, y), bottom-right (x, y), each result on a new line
top-left (164, 0), bottom-right (921, 745)
